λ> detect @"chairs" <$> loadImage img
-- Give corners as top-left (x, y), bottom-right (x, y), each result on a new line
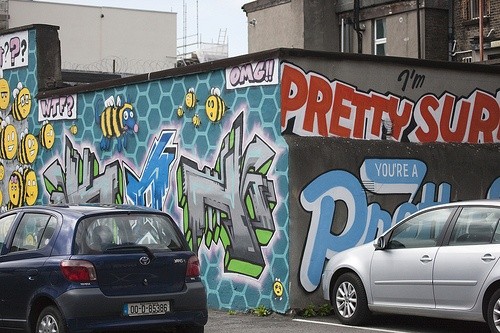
top-left (456, 217), bottom-right (495, 243)
top-left (22, 226), bottom-right (122, 253)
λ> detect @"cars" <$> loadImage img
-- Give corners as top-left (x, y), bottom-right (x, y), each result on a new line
top-left (321, 198), bottom-right (500, 332)
top-left (1, 203), bottom-right (207, 333)
